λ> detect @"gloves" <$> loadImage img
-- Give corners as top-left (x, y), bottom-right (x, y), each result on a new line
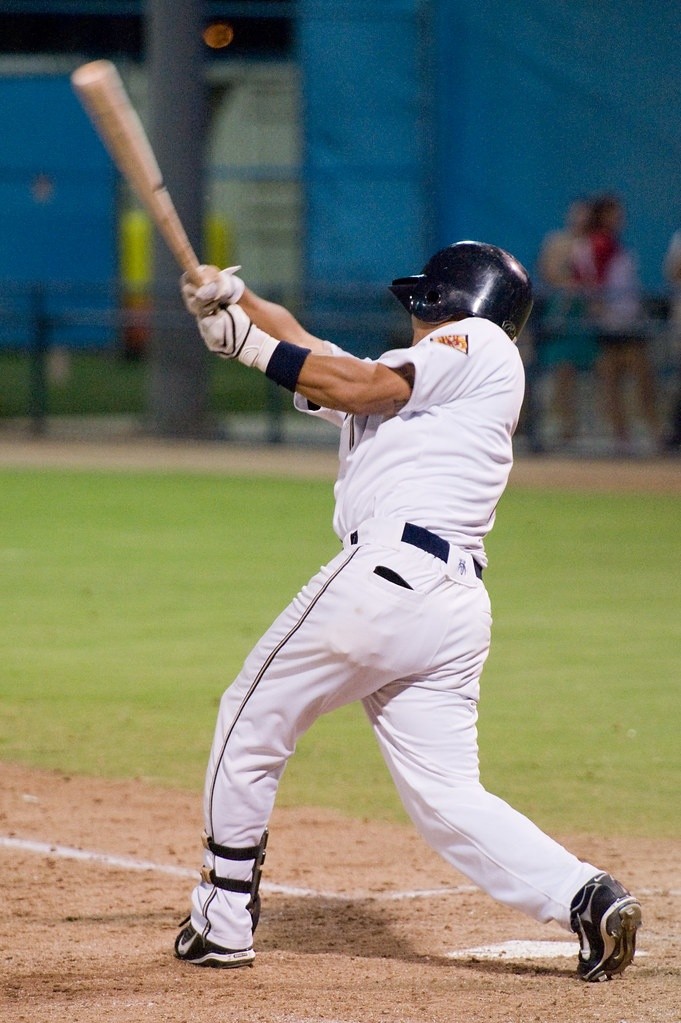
top-left (177, 263), bottom-right (243, 313)
top-left (194, 303), bottom-right (282, 374)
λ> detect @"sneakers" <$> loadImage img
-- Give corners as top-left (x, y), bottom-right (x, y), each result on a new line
top-left (570, 872), bottom-right (643, 983)
top-left (174, 914), bottom-right (258, 969)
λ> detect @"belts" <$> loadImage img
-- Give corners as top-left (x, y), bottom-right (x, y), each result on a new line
top-left (349, 521), bottom-right (488, 579)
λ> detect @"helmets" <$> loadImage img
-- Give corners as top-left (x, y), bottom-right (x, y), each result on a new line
top-left (388, 239), bottom-right (536, 340)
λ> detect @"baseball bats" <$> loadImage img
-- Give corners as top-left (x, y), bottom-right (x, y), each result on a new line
top-left (67, 58), bottom-right (209, 290)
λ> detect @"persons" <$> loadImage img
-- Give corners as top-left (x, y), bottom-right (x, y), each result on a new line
top-left (174, 240), bottom-right (645, 984)
top-left (541, 197), bottom-right (681, 458)
